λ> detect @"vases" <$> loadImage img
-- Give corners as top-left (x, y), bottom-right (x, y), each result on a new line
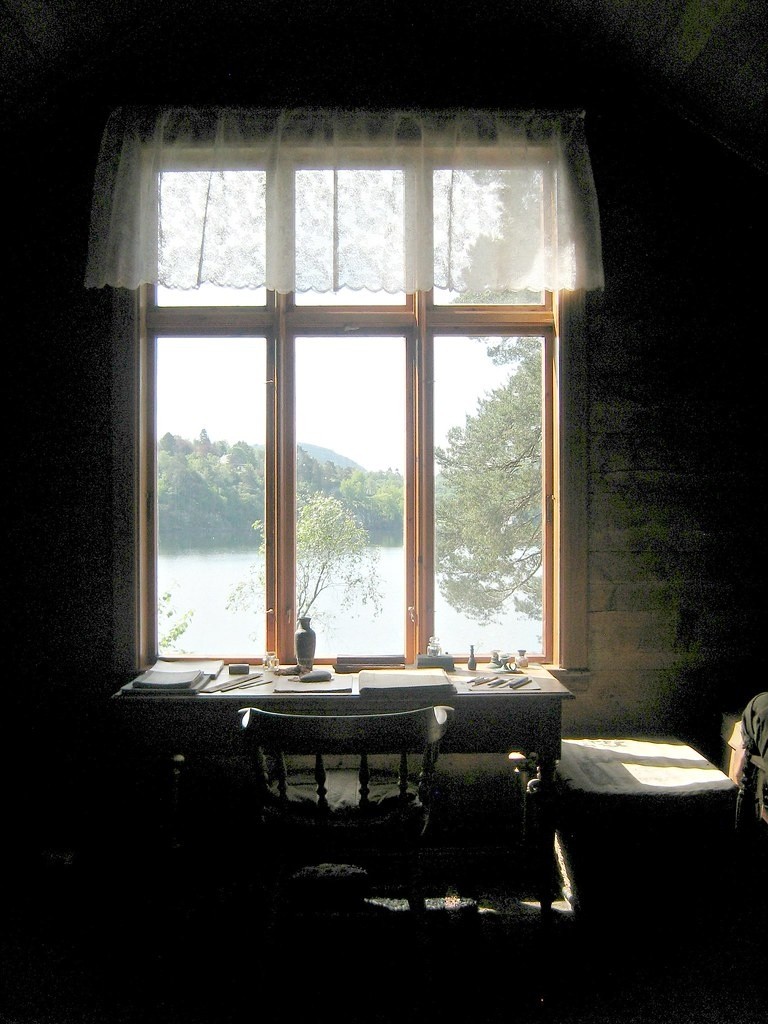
top-left (294, 617), bottom-right (316, 670)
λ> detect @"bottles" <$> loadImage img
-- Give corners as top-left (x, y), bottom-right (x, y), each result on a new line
top-left (515, 650), bottom-right (528, 668)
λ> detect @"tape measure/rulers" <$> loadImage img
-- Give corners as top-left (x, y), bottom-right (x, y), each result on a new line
top-left (199, 671), bottom-right (274, 696)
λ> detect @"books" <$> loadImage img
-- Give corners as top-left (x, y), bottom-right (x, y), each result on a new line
top-left (121, 658), bottom-right (225, 695)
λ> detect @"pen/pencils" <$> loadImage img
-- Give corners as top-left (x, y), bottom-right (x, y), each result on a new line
top-left (464, 673), bottom-right (536, 690)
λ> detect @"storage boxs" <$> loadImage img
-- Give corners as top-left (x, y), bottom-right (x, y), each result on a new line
top-left (552, 733), bottom-right (741, 921)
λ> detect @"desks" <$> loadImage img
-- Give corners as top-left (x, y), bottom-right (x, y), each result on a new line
top-left (112, 659), bottom-right (577, 925)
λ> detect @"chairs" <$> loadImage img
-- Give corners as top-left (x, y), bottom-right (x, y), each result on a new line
top-left (236, 703), bottom-right (452, 918)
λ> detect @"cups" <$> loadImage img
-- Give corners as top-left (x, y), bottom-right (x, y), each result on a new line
top-left (427, 645), bottom-right (442, 657)
top-left (262, 652), bottom-right (279, 672)
